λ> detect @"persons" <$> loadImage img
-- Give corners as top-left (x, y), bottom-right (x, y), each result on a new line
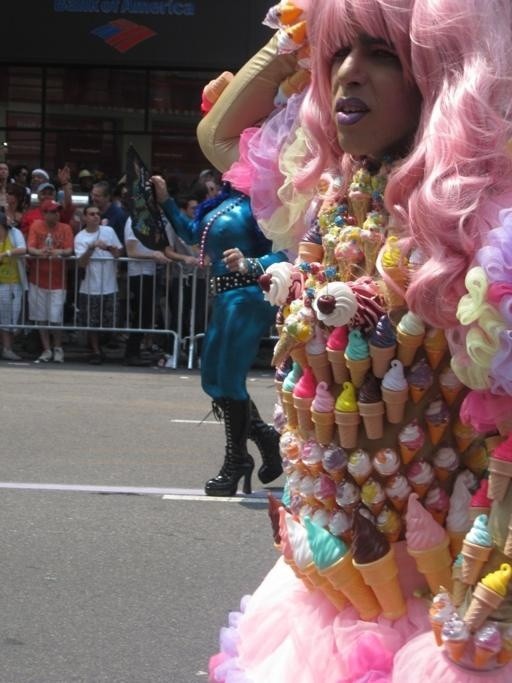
top-left (144, 165), bottom-right (286, 497)
top-left (1, 161), bottom-right (218, 364)
top-left (198, 0), bottom-right (512, 683)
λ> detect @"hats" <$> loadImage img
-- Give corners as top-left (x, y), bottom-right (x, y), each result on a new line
top-left (37, 181), bottom-right (64, 215)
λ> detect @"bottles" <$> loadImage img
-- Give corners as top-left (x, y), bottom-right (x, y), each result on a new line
top-left (45, 232), bottom-right (54, 252)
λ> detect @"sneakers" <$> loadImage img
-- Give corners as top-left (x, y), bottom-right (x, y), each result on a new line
top-left (37, 349), bottom-right (53, 363)
top-left (2, 347), bottom-right (23, 361)
top-left (52, 346), bottom-right (65, 365)
top-left (90, 345), bottom-right (183, 371)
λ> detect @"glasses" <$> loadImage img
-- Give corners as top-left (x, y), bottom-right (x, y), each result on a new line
top-left (89, 210), bottom-right (101, 217)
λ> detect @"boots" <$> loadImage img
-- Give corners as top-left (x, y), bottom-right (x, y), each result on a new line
top-left (203, 397), bottom-right (255, 498)
top-left (213, 392), bottom-right (285, 486)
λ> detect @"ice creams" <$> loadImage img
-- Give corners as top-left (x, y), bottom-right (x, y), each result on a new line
top-left (262, 4), bottom-right (307, 30)
top-left (200, 70), bottom-right (234, 113)
top-left (257, 169), bottom-right (512, 670)
top-left (276, 20), bottom-right (306, 56)
top-left (273, 68), bottom-right (310, 106)
